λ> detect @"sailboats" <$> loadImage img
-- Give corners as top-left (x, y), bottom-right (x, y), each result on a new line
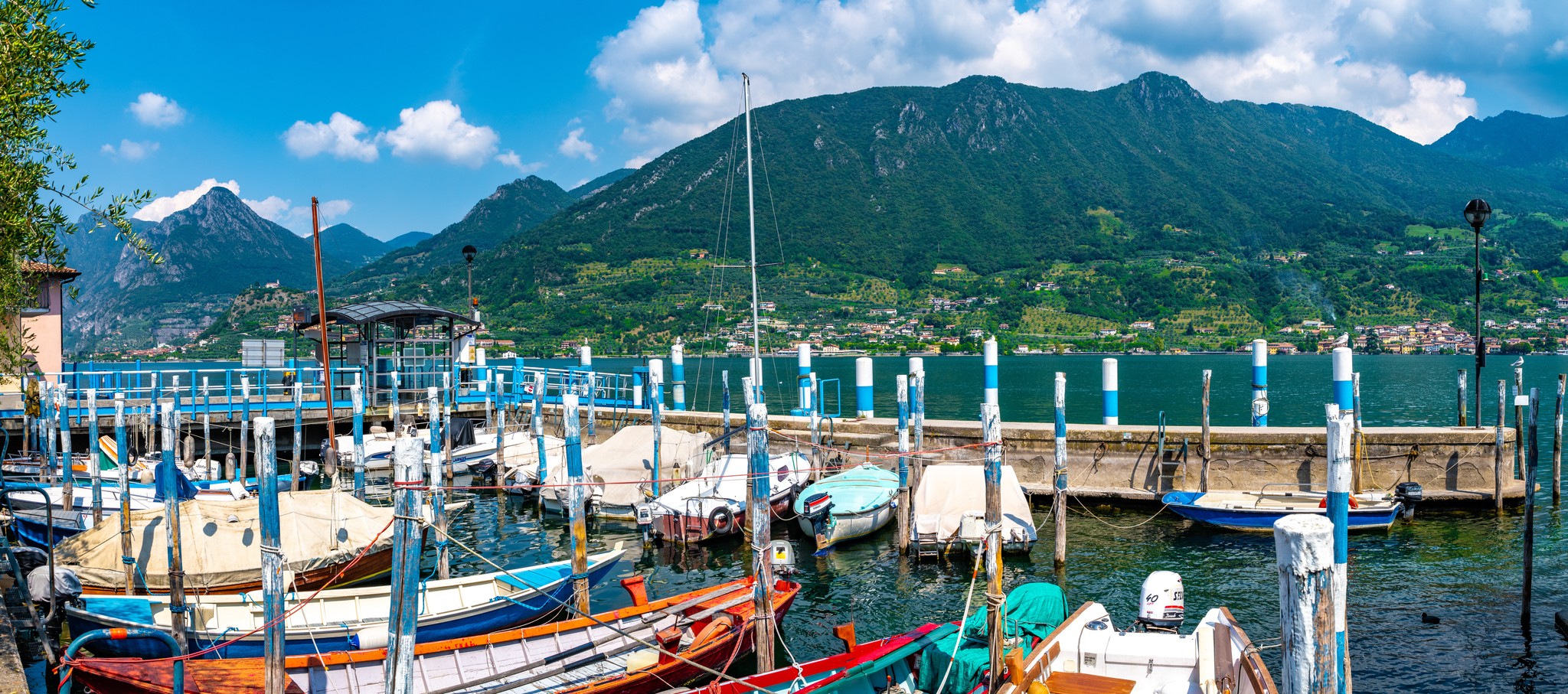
top-left (639, 77), bottom-right (813, 544)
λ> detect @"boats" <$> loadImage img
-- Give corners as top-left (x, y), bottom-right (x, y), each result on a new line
top-left (9, 486), bottom-right (476, 596)
top-left (0, 445), bottom-right (308, 546)
top-left (540, 426), bottom-right (715, 522)
top-left (319, 421), bottom-right (486, 474)
top-left (668, 582), bottom-right (1069, 694)
top-left (1162, 486), bottom-right (1402, 534)
top-left (422, 409), bottom-right (575, 496)
top-left (792, 461), bottom-right (901, 557)
top-left (60, 540), bottom-right (802, 694)
top-left (979, 571), bottom-right (1280, 694)
top-left (25, 542), bottom-right (628, 660)
top-left (909, 464), bottom-right (1038, 568)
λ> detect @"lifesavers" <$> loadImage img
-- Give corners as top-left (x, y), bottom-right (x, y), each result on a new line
top-left (127, 446), bottom-right (138, 466)
top-left (709, 506), bottom-right (733, 533)
top-left (790, 483), bottom-right (803, 501)
top-left (689, 617), bottom-right (731, 651)
top-left (46, 397), bottom-right (60, 423)
top-left (1319, 494), bottom-right (1357, 510)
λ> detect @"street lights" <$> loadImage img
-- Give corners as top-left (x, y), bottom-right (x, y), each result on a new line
top-left (1463, 199), bottom-right (1492, 430)
top-left (462, 245), bottom-right (477, 388)
